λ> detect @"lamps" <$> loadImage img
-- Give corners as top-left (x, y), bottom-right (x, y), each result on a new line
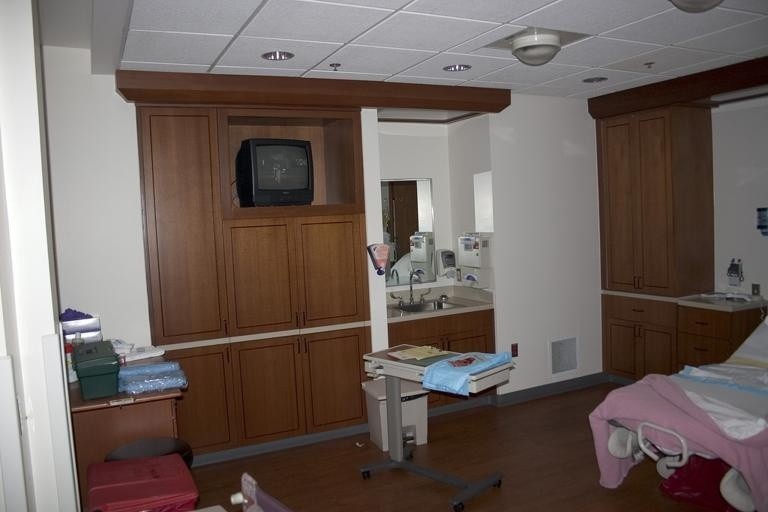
top-left (441, 62), bottom-right (473, 73)
top-left (509, 31), bottom-right (565, 67)
top-left (755, 205), bottom-right (768, 237)
top-left (260, 49), bottom-right (295, 64)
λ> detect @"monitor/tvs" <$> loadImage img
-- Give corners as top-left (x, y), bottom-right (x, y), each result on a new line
top-left (235, 137), bottom-right (314, 207)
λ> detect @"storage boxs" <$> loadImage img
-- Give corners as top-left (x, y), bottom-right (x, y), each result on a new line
top-left (410, 262), bottom-right (436, 284)
top-left (70, 340), bottom-right (120, 402)
top-left (83, 452), bottom-right (200, 512)
top-left (455, 230), bottom-right (491, 269)
top-left (457, 261), bottom-right (493, 291)
top-left (406, 231), bottom-right (435, 263)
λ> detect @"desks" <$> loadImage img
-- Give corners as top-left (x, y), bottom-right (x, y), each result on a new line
top-left (359, 341), bottom-right (515, 511)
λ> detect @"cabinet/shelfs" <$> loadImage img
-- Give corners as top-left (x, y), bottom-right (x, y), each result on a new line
top-left (593, 105), bottom-right (716, 300)
top-left (599, 295), bottom-right (676, 386)
top-left (221, 103), bottom-right (366, 333)
top-left (387, 310), bottom-right (496, 418)
top-left (234, 326), bottom-right (371, 459)
top-left (132, 106), bottom-right (232, 347)
top-left (166, 342), bottom-right (235, 469)
top-left (67, 379), bottom-right (183, 498)
top-left (677, 305), bottom-right (767, 373)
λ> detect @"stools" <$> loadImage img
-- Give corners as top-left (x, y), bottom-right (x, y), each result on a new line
top-left (104, 435), bottom-right (195, 476)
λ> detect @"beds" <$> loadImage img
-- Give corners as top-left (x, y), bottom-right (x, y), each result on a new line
top-left (587, 306), bottom-right (767, 512)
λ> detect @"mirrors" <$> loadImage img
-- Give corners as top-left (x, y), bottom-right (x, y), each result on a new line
top-left (380, 176), bottom-right (438, 287)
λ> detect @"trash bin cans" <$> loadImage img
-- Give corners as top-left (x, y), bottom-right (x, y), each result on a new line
top-left (360, 377), bottom-right (431, 452)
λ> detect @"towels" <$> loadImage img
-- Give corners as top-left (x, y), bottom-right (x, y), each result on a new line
top-left (368, 243), bottom-right (390, 276)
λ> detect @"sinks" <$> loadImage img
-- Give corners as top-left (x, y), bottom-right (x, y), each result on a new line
top-left (394, 300), bottom-right (464, 313)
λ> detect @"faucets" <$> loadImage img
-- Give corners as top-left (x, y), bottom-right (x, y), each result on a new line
top-left (391, 269), bottom-right (400, 285)
top-left (409, 273), bottom-right (422, 305)
